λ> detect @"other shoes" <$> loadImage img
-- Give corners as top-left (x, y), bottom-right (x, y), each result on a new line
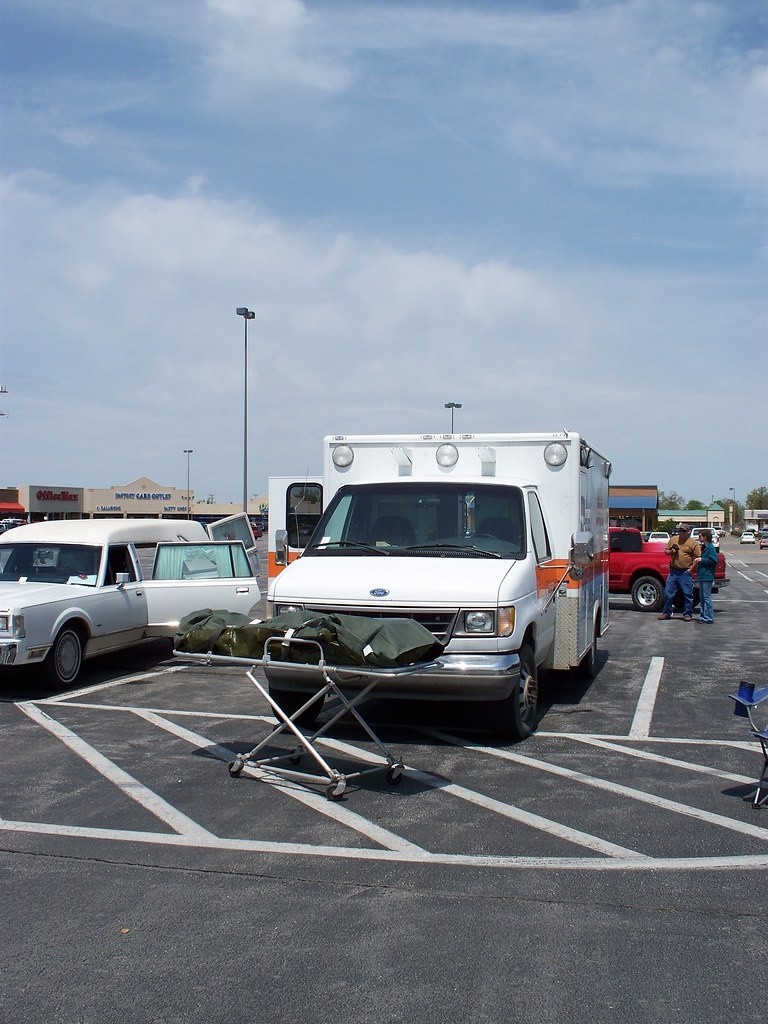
top-left (658, 613), bottom-right (671, 620)
top-left (695, 620), bottom-right (713, 624)
top-left (684, 616), bottom-right (692, 621)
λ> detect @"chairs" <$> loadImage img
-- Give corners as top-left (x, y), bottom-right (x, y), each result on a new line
top-left (370, 516), bottom-right (415, 545)
top-left (727, 685), bottom-right (768, 809)
top-left (475, 516), bottom-right (515, 544)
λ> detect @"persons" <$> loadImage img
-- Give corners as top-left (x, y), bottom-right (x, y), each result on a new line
top-left (695, 529), bottom-right (718, 624)
top-left (658, 524), bottom-right (700, 621)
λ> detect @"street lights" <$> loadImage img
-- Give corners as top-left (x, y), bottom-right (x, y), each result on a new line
top-left (729, 488), bottom-right (735, 534)
top-left (235, 306), bottom-right (255, 512)
top-left (183, 449), bottom-right (194, 520)
top-left (443, 403), bottom-right (462, 433)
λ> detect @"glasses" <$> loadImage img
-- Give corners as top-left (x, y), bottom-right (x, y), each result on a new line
top-left (699, 535), bottom-right (704, 537)
top-left (679, 530), bottom-right (686, 532)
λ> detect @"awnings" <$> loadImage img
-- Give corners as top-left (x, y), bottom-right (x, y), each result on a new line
top-left (0, 502), bottom-right (24, 512)
top-left (609, 496), bottom-right (657, 509)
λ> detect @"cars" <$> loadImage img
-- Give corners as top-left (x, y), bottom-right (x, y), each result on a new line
top-left (740, 532), bottom-right (755, 544)
top-left (691, 527), bottom-right (719, 553)
top-left (759, 536), bottom-right (768, 549)
top-left (647, 531), bottom-right (670, 543)
top-left (0, 511), bottom-right (263, 690)
top-left (758, 528), bottom-right (768, 535)
top-left (250, 523), bottom-right (262, 540)
top-left (714, 526), bottom-right (726, 537)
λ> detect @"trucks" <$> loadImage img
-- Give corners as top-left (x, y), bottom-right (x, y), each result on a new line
top-left (266, 429), bottom-right (612, 740)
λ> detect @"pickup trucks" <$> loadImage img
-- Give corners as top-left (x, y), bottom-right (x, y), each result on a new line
top-left (608, 526), bottom-right (731, 613)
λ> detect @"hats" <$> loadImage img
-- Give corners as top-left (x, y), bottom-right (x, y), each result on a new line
top-left (678, 523), bottom-right (690, 531)
